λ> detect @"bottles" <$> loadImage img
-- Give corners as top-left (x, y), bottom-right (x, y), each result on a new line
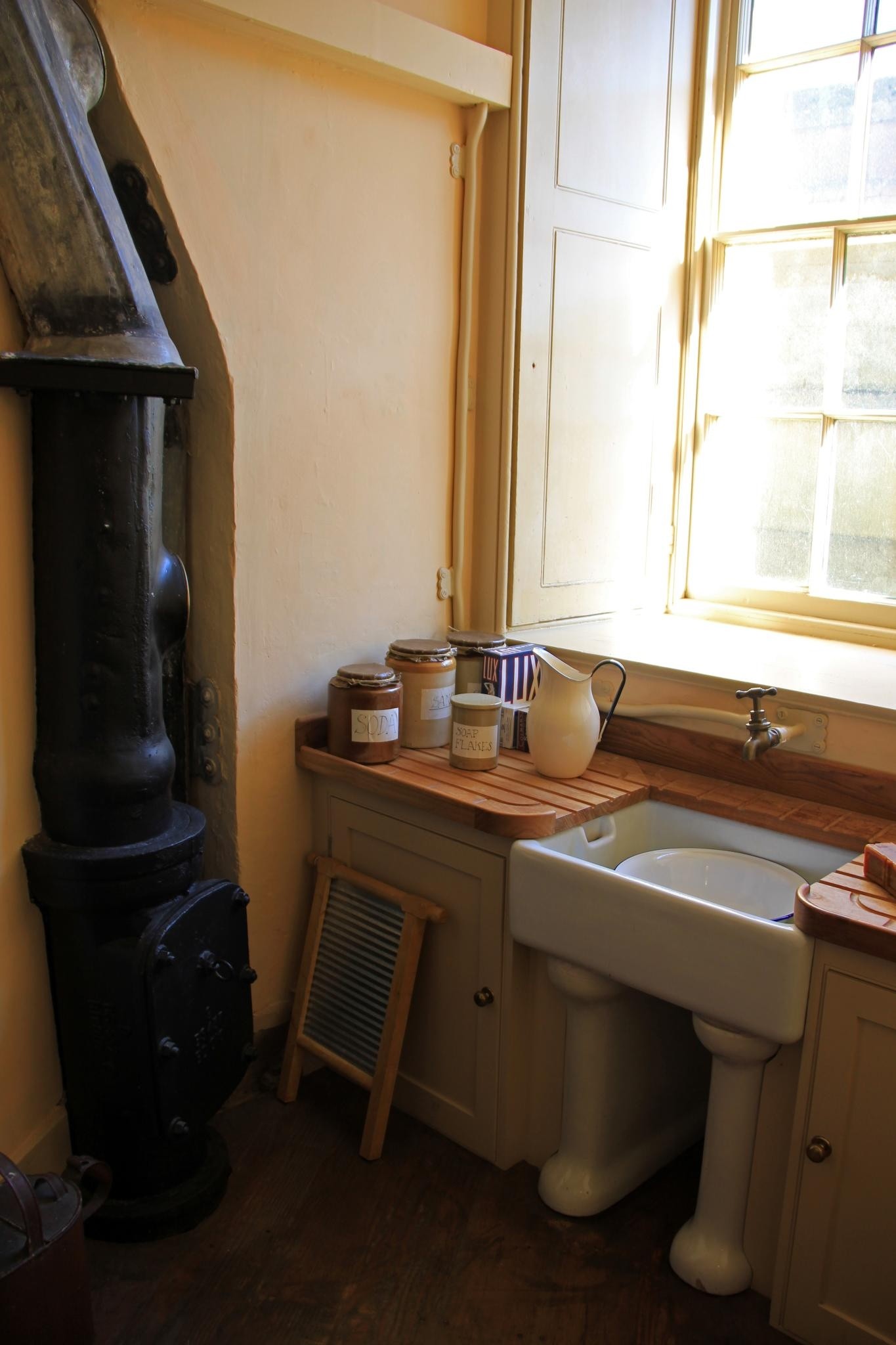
top-left (327, 663), bottom-right (403, 764)
top-left (449, 693), bottom-right (503, 770)
top-left (446, 631), bottom-right (507, 694)
top-left (385, 639), bottom-right (457, 748)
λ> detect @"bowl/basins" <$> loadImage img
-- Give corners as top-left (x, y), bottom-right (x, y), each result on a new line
top-left (614, 846), bottom-right (809, 923)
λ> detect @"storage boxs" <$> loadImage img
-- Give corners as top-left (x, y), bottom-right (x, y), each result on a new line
top-left (483, 642), bottom-right (546, 752)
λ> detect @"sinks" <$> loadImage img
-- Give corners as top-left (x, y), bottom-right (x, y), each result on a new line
top-left (507, 798), bottom-right (865, 1044)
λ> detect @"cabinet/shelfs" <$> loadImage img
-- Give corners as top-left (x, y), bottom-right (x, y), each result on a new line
top-left (278, 776), bottom-right (509, 1175)
top-left (760, 949), bottom-right (896, 1344)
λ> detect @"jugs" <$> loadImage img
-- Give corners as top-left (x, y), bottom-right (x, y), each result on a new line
top-left (526, 647), bottom-right (626, 777)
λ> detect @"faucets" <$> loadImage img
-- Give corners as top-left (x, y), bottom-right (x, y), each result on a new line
top-left (735, 687), bottom-right (786, 762)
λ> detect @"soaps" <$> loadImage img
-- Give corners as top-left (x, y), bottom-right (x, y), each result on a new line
top-left (863, 843), bottom-right (896, 899)
